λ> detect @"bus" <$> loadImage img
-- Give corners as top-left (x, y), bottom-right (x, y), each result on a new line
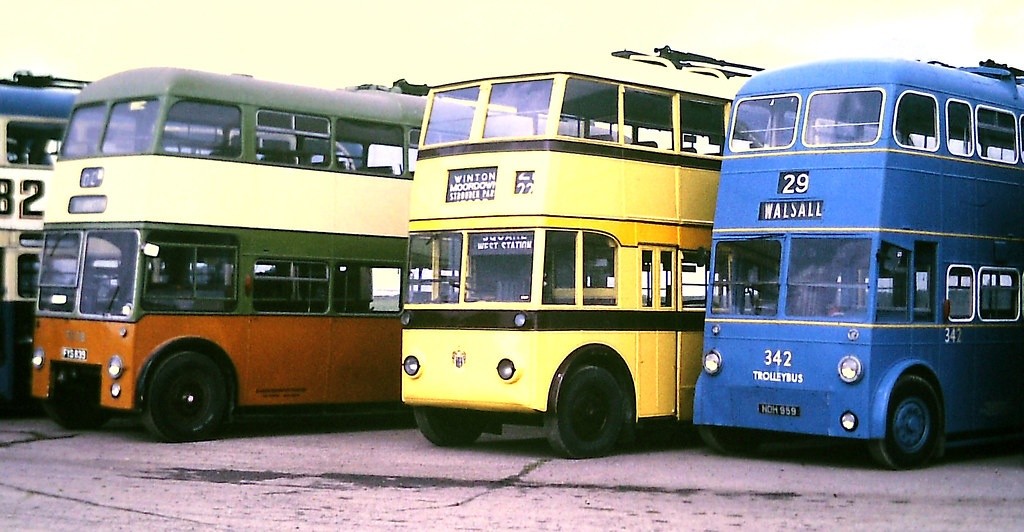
top-left (0, 79), bottom-right (94, 414)
top-left (400, 45), bottom-right (764, 461)
top-left (30, 65), bottom-right (426, 445)
top-left (689, 60), bottom-right (1023, 469)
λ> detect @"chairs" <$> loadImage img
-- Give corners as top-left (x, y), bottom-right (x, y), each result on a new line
top-left (59, 115), bottom-right (714, 318)
